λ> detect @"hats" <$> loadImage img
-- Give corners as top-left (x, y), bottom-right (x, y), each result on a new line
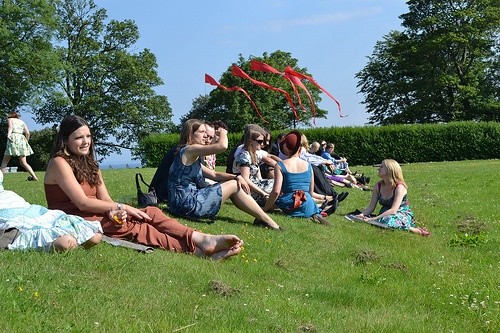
top-left (279, 131), bottom-right (301, 156)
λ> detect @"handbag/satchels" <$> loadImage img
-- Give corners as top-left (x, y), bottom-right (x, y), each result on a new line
top-left (136, 172), bottom-right (158, 206)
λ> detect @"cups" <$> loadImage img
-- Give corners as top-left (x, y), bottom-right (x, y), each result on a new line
top-left (114, 204), bottom-right (125, 227)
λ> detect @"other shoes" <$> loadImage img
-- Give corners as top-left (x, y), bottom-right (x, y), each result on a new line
top-left (339, 192), bottom-right (348, 202)
top-left (327, 195), bottom-right (339, 206)
top-left (253, 218), bottom-right (287, 232)
top-left (352, 171), bottom-right (357, 176)
top-left (357, 172), bottom-right (370, 185)
top-left (324, 200), bottom-right (339, 216)
top-left (314, 214), bottom-right (334, 227)
top-left (25, 176), bottom-right (38, 181)
top-left (319, 199), bottom-right (328, 209)
top-left (361, 188), bottom-right (373, 191)
top-left (419, 227), bottom-right (431, 236)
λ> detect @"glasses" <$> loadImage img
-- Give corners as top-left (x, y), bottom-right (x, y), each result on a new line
top-left (251, 136), bottom-right (264, 144)
top-left (329, 146), bottom-right (334, 148)
top-left (322, 143), bottom-right (326, 146)
